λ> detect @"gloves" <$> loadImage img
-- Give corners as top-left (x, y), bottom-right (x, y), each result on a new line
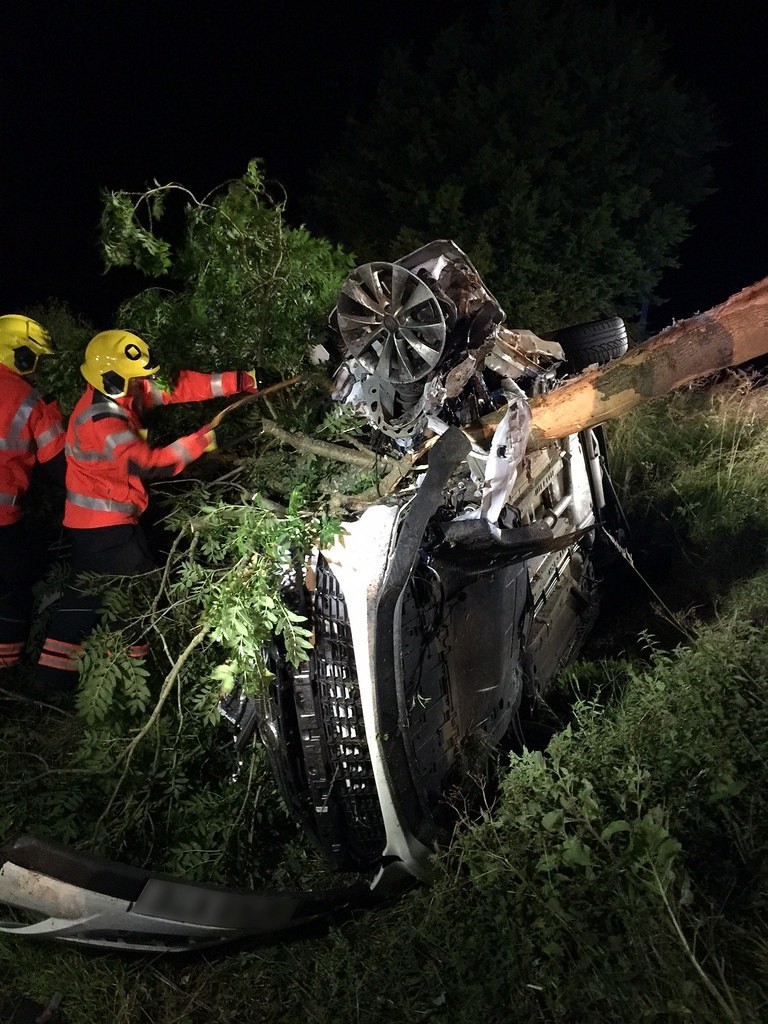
top-left (202, 416), bottom-right (225, 453)
top-left (239, 367), bottom-right (282, 394)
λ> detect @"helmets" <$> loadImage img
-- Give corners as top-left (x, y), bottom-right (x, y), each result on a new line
top-left (80, 329), bottom-right (160, 399)
top-left (0, 314), bottom-right (58, 377)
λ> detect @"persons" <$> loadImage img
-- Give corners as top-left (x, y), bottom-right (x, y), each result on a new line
top-left (0, 314), bottom-right (67, 718)
top-left (35, 329), bottom-right (278, 718)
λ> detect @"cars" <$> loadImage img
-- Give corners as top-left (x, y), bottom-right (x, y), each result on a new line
top-left (0, 314), bottom-right (636, 951)
